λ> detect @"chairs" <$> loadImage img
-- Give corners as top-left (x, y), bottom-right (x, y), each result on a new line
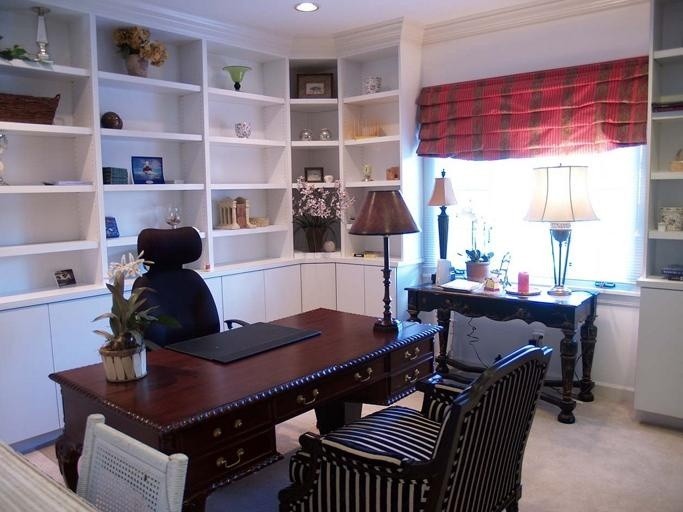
top-left (287, 344), bottom-right (553, 509)
top-left (76, 412), bottom-right (189, 512)
top-left (130, 226), bottom-right (251, 351)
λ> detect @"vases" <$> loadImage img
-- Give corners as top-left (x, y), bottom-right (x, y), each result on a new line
top-left (126, 54), bottom-right (149, 78)
top-left (98, 343), bottom-right (148, 383)
top-left (466, 261), bottom-right (490, 282)
top-left (302, 226), bottom-right (328, 252)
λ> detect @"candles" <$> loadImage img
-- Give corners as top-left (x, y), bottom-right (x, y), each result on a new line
top-left (518, 272), bottom-right (529, 293)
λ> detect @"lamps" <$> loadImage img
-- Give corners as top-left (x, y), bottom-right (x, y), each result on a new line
top-left (524, 162), bottom-right (599, 296)
top-left (349, 188), bottom-right (422, 330)
top-left (222, 66), bottom-right (249, 91)
top-left (426, 168), bottom-right (457, 280)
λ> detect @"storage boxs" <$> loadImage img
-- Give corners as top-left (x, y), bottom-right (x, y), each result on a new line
top-left (103, 166), bottom-right (129, 185)
top-left (659, 206), bottom-right (683, 232)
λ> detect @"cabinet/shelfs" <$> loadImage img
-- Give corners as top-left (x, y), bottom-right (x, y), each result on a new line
top-left (634, 0), bottom-right (682, 430)
top-left (0, 1), bottom-right (426, 456)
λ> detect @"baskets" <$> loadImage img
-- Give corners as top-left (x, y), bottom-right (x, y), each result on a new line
top-left (0, 93), bottom-right (60, 124)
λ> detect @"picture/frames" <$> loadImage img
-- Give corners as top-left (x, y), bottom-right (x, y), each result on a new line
top-left (305, 167), bottom-right (324, 183)
top-left (296, 73), bottom-right (333, 98)
top-left (53, 268), bottom-right (79, 289)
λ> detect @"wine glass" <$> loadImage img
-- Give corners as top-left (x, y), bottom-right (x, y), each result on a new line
top-left (165, 206), bottom-right (181, 228)
top-left (0, 135), bottom-right (10, 185)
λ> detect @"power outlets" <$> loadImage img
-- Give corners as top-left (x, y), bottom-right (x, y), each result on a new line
top-left (532, 332), bottom-right (545, 346)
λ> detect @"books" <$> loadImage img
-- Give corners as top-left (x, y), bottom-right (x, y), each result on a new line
top-left (437, 276), bottom-right (483, 295)
top-left (650, 93), bottom-right (682, 113)
top-left (660, 263), bottom-right (683, 281)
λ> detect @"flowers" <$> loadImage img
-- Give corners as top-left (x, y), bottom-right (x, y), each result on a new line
top-left (292, 175), bottom-right (356, 243)
top-left (455, 199), bottom-right (495, 262)
top-left (91, 248), bottom-right (161, 347)
top-left (112, 25), bottom-right (169, 68)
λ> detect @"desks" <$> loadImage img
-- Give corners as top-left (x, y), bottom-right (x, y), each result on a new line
top-left (49, 308), bottom-right (444, 512)
top-left (0, 439), bottom-right (101, 512)
top-left (404, 279), bottom-right (600, 423)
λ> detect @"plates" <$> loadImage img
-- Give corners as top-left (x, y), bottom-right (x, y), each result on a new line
top-left (513, 289), bottom-right (538, 293)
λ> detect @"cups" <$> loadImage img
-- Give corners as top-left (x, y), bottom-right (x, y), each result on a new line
top-left (369, 76), bottom-right (384, 93)
top-left (298, 129), bottom-right (335, 142)
top-left (235, 123), bottom-right (254, 143)
top-left (518, 275), bottom-right (528, 291)
